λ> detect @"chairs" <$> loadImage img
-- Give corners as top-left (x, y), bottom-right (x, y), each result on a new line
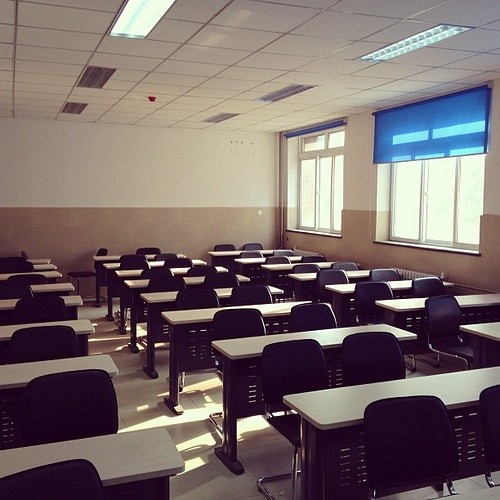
top-left (0, 243), bottom-right (500, 500)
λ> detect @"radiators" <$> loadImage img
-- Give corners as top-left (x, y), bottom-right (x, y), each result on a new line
top-left (391, 267), bottom-right (445, 283)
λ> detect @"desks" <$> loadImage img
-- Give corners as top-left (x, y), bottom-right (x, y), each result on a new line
top-left (461, 322), bottom-right (500, 369)
top-left (102, 259), bottom-right (207, 321)
top-left (0, 319), bottom-right (95, 358)
top-left (93, 251), bottom-right (187, 308)
top-left (26, 258), bottom-right (51, 264)
top-left (121, 274), bottom-right (250, 352)
top-left (325, 278), bottom-right (454, 328)
top-left (375, 292), bottom-right (499, 371)
top-left (0, 295), bottom-right (83, 320)
top-left (0, 355), bottom-right (119, 391)
top-left (283, 366), bottom-right (500, 499)
top-left (288, 269), bottom-right (371, 302)
top-left (261, 262), bottom-right (335, 286)
top-left (116, 266), bottom-right (229, 334)
top-left (234, 255), bottom-right (302, 278)
top-left (207, 249), bottom-right (294, 268)
top-left (210, 324), bottom-right (416, 476)
top-left (28, 283), bottom-right (75, 297)
top-left (137, 285), bottom-right (284, 379)
top-left (33, 263), bottom-right (57, 271)
top-left (161, 301), bottom-right (312, 416)
top-left (0, 427), bottom-right (185, 500)
top-left (0, 270), bottom-right (62, 284)
